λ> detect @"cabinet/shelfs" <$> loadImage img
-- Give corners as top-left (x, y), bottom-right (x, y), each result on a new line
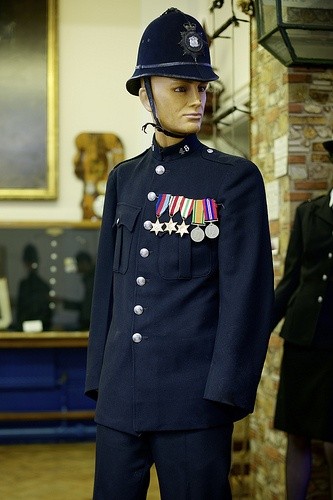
top-left (0, 340), bottom-right (97, 445)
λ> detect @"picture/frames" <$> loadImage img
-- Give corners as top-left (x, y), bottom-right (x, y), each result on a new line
top-left (0, 0), bottom-right (58, 201)
top-left (0, 221), bottom-right (104, 340)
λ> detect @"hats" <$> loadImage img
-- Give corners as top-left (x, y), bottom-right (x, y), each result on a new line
top-left (125, 7), bottom-right (219, 96)
top-left (322, 126), bottom-right (333, 151)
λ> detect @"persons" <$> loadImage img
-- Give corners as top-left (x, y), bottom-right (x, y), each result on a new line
top-left (84, 8), bottom-right (276, 500)
top-left (271, 140), bottom-right (333, 500)
